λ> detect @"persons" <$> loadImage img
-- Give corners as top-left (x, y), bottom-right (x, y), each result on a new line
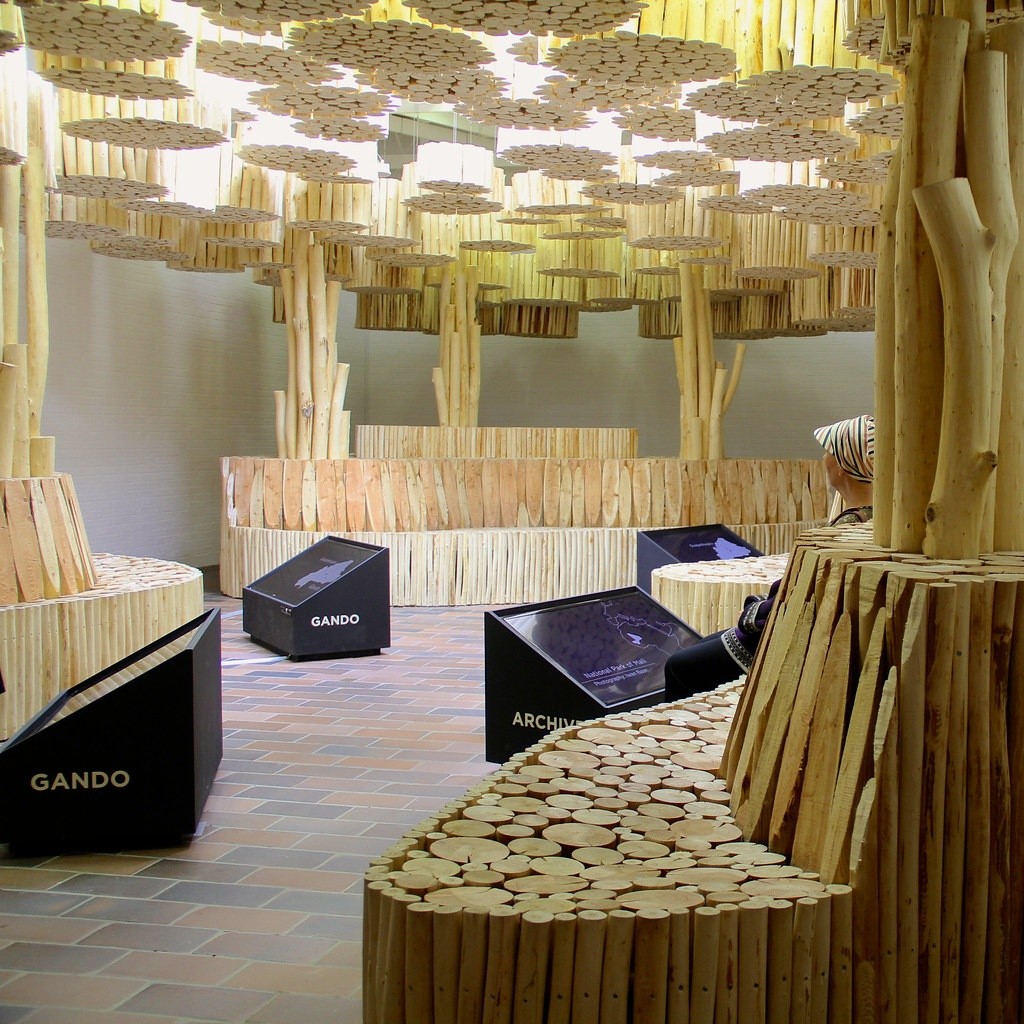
top-left (663, 414), bottom-right (878, 703)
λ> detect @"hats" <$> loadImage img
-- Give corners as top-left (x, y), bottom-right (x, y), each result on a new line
top-left (813, 414), bottom-right (875, 484)
